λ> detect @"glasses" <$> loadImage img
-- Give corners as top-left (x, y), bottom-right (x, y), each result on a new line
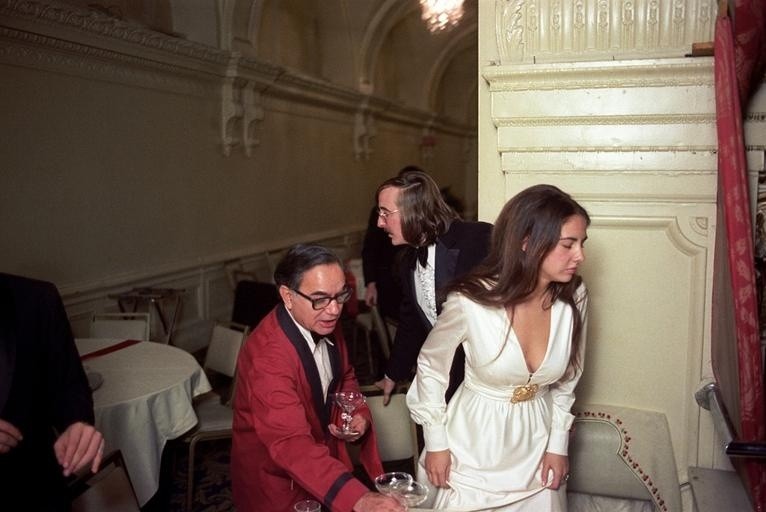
top-left (378, 208), bottom-right (399, 217)
top-left (292, 288), bottom-right (351, 310)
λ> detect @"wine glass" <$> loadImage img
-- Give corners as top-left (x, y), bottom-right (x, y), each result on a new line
top-left (332, 392), bottom-right (367, 439)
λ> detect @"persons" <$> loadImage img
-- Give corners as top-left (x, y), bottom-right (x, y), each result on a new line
top-left (401, 183), bottom-right (593, 512)
top-left (0, 262), bottom-right (108, 510)
top-left (361, 170), bottom-right (499, 407)
top-left (228, 242), bottom-right (410, 512)
top-left (361, 163), bottom-right (427, 331)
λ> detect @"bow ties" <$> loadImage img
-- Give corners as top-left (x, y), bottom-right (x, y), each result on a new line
top-left (406, 246), bottom-right (429, 270)
top-left (310, 331), bottom-right (336, 345)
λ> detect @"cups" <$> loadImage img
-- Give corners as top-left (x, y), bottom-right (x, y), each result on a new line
top-left (294, 501), bottom-right (321, 512)
top-left (375, 470), bottom-right (429, 512)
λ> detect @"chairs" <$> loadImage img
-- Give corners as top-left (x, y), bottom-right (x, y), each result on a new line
top-left (86, 311), bottom-right (153, 344)
top-left (74, 450), bottom-right (153, 512)
top-left (365, 380), bottom-right (420, 469)
top-left (177, 319), bottom-right (252, 510)
top-left (221, 245), bottom-right (395, 386)
